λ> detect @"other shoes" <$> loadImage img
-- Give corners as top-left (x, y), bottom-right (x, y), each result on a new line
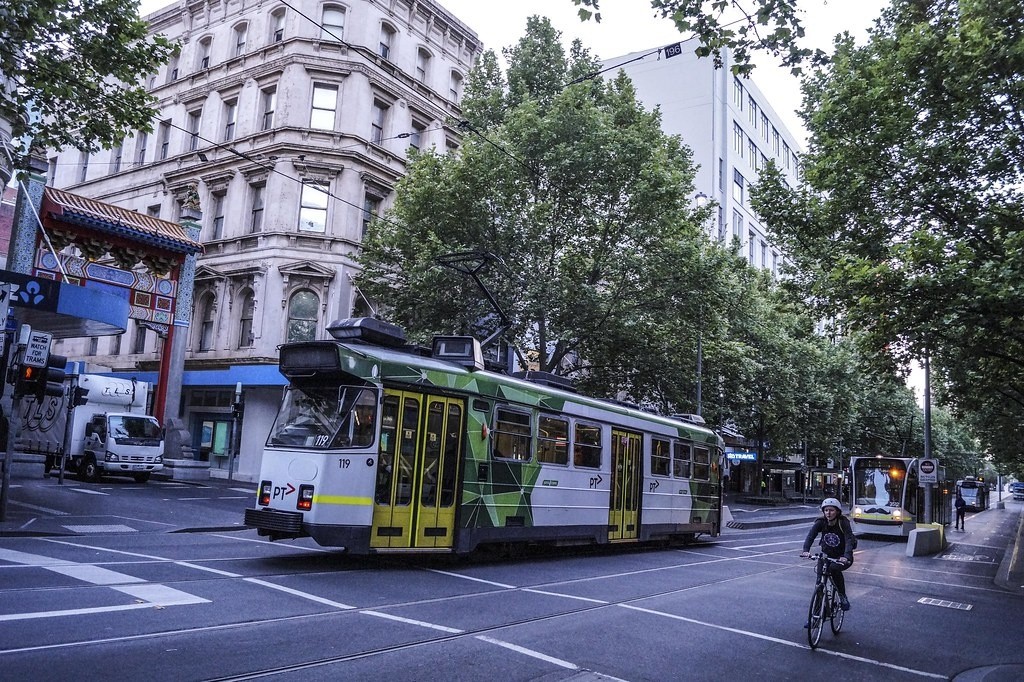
top-left (961, 528), bottom-right (964, 529)
top-left (955, 527), bottom-right (958, 529)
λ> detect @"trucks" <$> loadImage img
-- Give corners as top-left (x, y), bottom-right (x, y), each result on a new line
top-left (10, 372), bottom-right (168, 484)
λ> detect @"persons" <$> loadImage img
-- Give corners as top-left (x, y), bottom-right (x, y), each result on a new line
top-left (318, 396), bottom-right (362, 446)
top-left (761, 479), bottom-right (766, 494)
top-left (0, 404), bottom-right (9, 452)
top-left (94, 425), bottom-right (105, 443)
top-left (583, 427), bottom-right (601, 466)
top-left (574, 447), bottom-right (586, 467)
top-left (955, 493), bottom-right (966, 531)
top-left (802, 498), bottom-right (854, 628)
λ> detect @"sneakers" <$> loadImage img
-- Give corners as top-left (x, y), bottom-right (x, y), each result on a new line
top-left (840, 595), bottom-right (849, 610)
top-left (804, 616), bottom-right (818, 629)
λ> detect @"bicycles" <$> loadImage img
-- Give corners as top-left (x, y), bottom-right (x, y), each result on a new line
top-left (799, 552), bottom-right (847, 649)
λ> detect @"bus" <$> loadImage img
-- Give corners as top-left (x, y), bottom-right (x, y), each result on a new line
top-left (242, 251), bottom-right (731, 566)
top-left (841, 414), bottom-right (954, 540)
top-left (955, 467), bottom-right (990, 512)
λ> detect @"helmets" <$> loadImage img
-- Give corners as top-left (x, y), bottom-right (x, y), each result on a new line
top-left (821, 498), bottom-right (842, 511)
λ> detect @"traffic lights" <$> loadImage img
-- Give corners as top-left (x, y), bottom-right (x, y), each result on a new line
top-left (40, 352), bottom-right (68, 396)
top-left (18, 365), bottom-right (41, 395)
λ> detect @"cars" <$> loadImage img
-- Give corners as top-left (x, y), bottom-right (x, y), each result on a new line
top-left (824, 483), bottom-right (835, 494)
top-left (989, 486), bottom-right (996, 491)
top-left (1008, 482), bottom-right (1024, 501)
